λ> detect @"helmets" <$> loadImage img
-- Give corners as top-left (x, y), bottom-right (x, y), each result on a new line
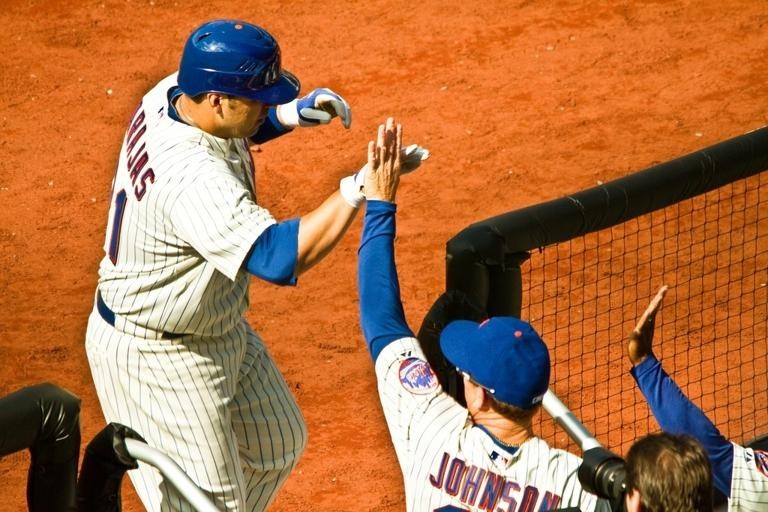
top-left (177, 19), bottom-right (300, 105)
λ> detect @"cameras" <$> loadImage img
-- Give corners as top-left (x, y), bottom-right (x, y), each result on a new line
top-left (576, 446), bottom-right (630, 501)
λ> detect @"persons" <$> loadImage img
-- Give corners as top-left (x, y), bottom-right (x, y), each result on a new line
top-left (625, 283), bottom-right (768, 511)
top-left (355, 115), bottom-right (612, 511)
top-left (618, 431), bottom-right (714, 511)
top-left (85, 19), bottom-right (431, 512)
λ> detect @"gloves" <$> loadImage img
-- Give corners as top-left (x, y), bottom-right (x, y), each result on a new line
top-left (278, 88), bottom-right (352, 130)
top-left (339, 144), bottom-right (423, 209)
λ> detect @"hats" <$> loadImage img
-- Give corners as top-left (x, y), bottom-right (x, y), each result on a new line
top-left (439, 315), bottom-right (550, 409)
